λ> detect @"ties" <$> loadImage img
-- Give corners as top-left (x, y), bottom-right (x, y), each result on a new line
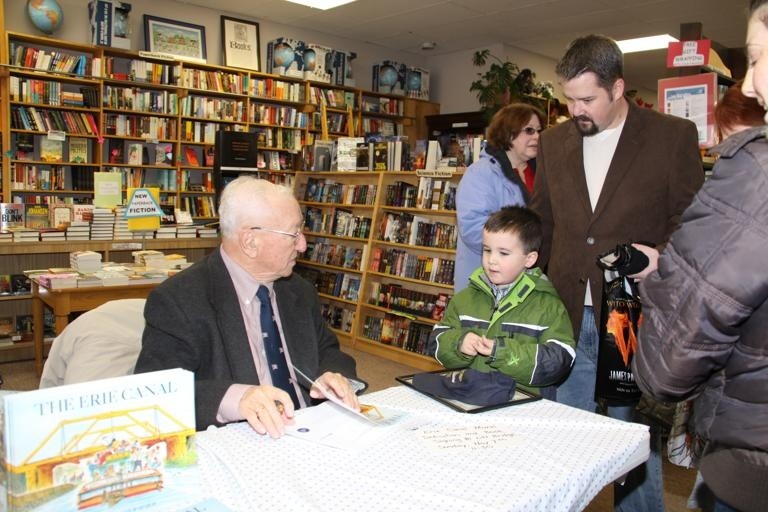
top-left (255, 284), bottom-right (301, 410)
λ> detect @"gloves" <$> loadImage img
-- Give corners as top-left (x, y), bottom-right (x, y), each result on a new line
top-left (596, 239), bottom-right (656, 276)
top-left (634, 391), bottom-right (676, 452)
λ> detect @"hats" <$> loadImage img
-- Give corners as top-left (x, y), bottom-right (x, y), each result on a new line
top-left (412, 369), bottom-right (516, 408)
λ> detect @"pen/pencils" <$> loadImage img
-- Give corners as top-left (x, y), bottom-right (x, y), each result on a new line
top-left (275, 401), bottom-right (283, 415)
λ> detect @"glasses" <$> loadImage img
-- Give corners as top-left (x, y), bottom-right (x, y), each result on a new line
top-left (518, 127), bottom-right (542, 135)
top-left (251, 220), bottom-right (306, 243)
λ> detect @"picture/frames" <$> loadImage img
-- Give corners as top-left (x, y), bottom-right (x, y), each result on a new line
top-left (143, 13), bottom-right (206, 65)
top-left (394, 367), bottom-right (543, 413)
top-left (219, 14), bottom-right (262, 72)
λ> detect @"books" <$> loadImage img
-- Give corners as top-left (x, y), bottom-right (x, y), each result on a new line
top-left (104, 55), bottom-right (249, 219)
top-left (279, 359), bottom-right (386, 450)
top-left (0, 367), bottom-right (203, 512)
top-left (296, 134), bottom-right (483, 355)
top-left (250, 76), bottom-right (406, 172)
top-left (0, 203), bottom-right (218, 341)
top-left (9, 44), bottom-right (102, 209)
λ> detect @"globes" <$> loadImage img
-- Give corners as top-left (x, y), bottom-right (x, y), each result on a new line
top-left (27, 0), bottom-right (63, 35)
top-left (274, 43), bottom-right (295, 67)
top-left (380, 65), bottom-right (399, 86)
top-left (407, 71), bottom-right (420, 90)
top-left (303, 49), bottom-right (315, 71)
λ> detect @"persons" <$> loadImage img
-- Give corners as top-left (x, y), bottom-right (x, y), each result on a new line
top-left (428, 205), bottom-right (577, 402)
top-left (133, 170), bottom-right (373, 439)
top-left (627, 1), bottom-right (767, 512)
top-left (450, 101), bottom-right (542, 296)
top-left (526, 34), bottom-right (706, 512)
top-left (712, 80), bottom-right (767, 148)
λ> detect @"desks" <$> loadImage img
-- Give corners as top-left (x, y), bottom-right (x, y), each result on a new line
top-left (0, 366), bottom-right (650, 512)
